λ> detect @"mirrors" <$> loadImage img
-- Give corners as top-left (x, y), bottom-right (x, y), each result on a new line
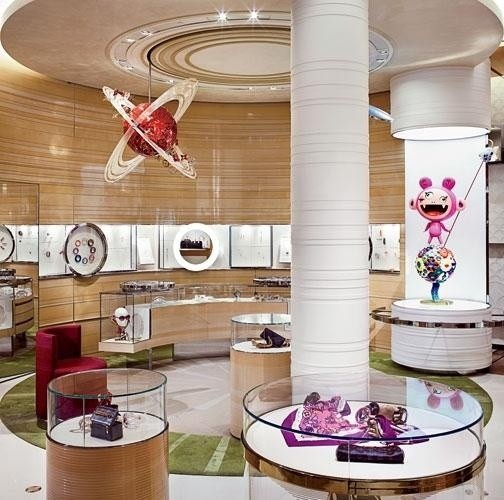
top-left (174, 223), bottom-right (218, 273)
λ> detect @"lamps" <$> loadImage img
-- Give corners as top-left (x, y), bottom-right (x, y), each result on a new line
top-left (102, 62), bottom-right (200, 182)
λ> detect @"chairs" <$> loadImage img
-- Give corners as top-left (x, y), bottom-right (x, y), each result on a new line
top-left (34, 325), bottom-right (107, 431)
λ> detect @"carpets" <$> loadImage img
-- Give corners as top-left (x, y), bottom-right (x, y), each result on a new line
top-left (0, 327), bottom-right (493, 477)
